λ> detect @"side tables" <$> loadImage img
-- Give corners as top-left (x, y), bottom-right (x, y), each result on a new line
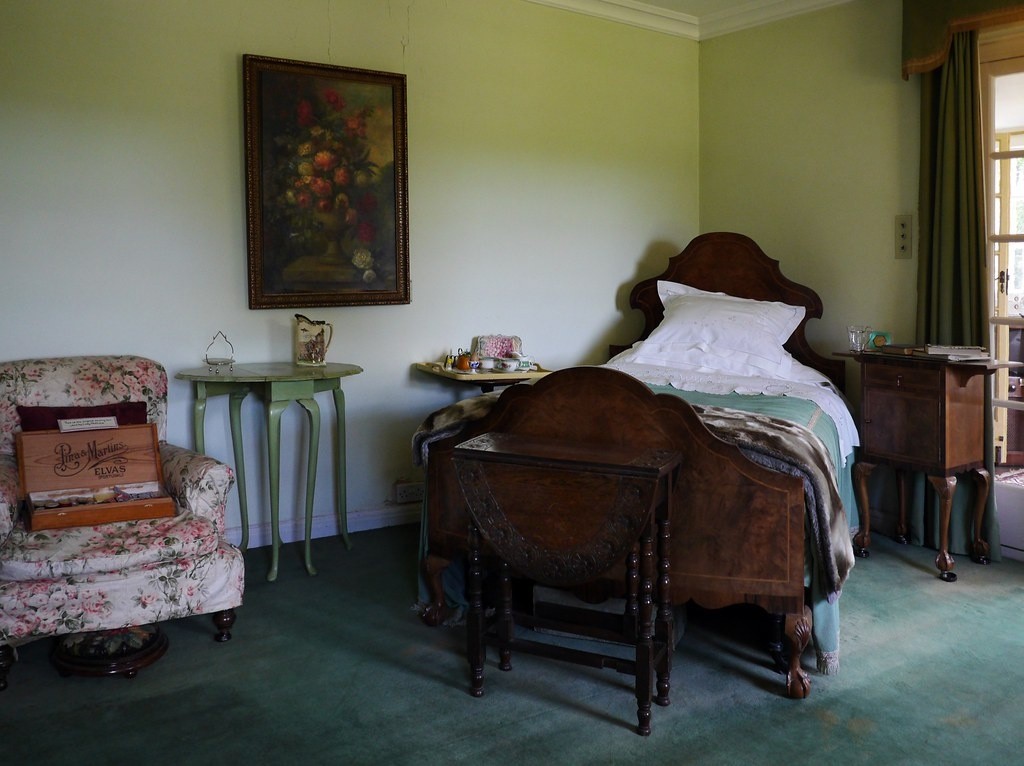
top-left (417, 359), bottom-right (556, 394)
top-left (174, 360), bottom-right (365, 582)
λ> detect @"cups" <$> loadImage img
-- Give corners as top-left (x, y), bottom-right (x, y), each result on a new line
top-left (847, 324), bottom-right (874, 351)
top-left (468, 360), bottom-right (479, 375)
top-left (501, 356), bottom-right (522, 373)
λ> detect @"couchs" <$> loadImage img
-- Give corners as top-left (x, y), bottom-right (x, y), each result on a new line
top-left (0, 355), bottom-right (245, 691)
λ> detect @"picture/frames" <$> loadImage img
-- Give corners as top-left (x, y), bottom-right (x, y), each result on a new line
top-left (243, 51), bottom-right (412, 310)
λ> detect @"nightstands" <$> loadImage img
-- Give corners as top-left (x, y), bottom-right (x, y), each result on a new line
top-left (831, 342), bottom-right (1024, 582)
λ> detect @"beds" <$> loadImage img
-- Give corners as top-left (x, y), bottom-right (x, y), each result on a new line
top-left (411, 232), bottom-right (859, 702)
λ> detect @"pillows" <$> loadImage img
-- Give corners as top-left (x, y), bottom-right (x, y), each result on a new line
top-left (627, 281), bottom-right (806, 380)
top-left (16, 400), bottom-right (148, 433)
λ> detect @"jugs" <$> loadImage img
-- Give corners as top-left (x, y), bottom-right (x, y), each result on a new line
top-left (294, 312), bottom-right (333, 367)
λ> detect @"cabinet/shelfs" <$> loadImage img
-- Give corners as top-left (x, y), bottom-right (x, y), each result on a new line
top-left (453, 431), bottom-right (674, 737)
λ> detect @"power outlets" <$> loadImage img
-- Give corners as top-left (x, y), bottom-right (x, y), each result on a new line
top-left (396, 482), bottom-right (424, 504)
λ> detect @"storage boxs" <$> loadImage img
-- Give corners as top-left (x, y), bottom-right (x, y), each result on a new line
top-left (17, 422), bottom-right (178, 533)
